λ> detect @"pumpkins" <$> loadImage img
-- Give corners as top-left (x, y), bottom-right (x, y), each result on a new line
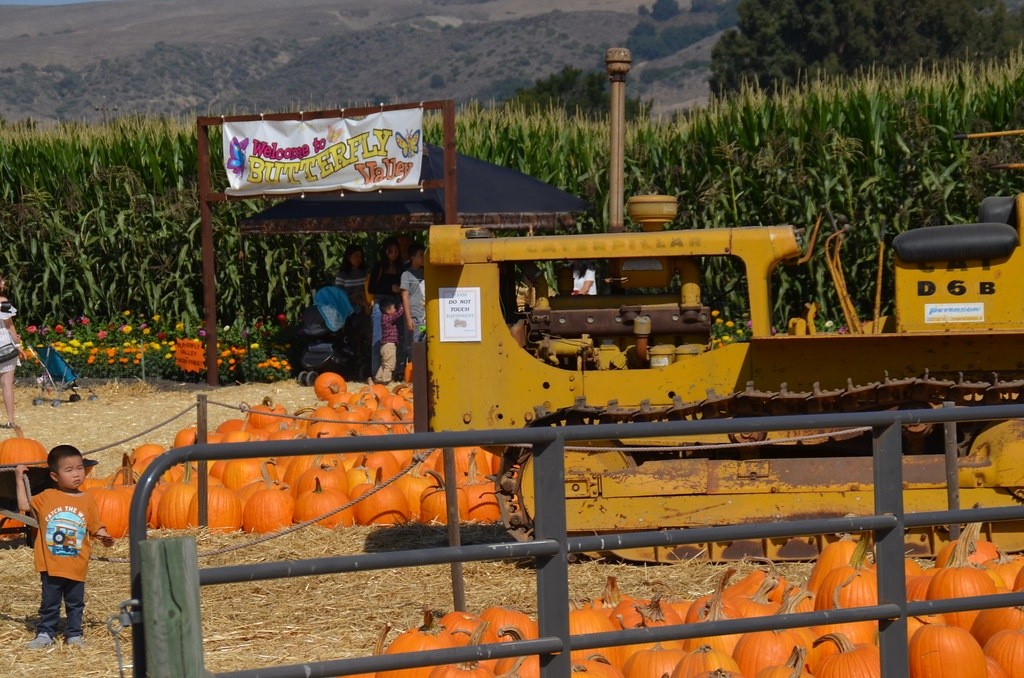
top-left (335, 504), bottom-right (1024, 678)
top-left (0, 360), bottom-right (516, 543)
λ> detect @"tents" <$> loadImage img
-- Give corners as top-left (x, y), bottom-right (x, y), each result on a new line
top-left (237, 126), bottom-right (586, 380)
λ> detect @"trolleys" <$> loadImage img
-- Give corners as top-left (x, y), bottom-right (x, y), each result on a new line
top-left (0, 459), bottom-right (99, 549)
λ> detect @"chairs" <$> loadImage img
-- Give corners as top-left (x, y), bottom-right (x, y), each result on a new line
top-left (892, 194), bottom-right (1017, 334)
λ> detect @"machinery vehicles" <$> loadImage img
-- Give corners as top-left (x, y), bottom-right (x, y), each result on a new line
top-left (413, 48), bottom-right (1022, 564)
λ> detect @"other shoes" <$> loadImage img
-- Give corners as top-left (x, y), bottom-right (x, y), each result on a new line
top-left (28, 633), bottom-right (55, 649)
top-left (66, 636), bottom-right (83, 646)
top-left (5, 422), bottom-right (17, 429)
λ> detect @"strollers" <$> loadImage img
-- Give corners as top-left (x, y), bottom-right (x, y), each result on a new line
top-left (20, 326), bottom-right (98, 407)
top-left (297, 286), bottom-right (373, 388)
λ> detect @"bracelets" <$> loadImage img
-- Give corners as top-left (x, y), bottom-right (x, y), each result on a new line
top-left (14, 338), bottom-right (21, 348)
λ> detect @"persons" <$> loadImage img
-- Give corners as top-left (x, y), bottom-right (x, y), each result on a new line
top-left (571, 259), bottom-right (597, 297)
top-left (291, 238), bottom-right (431, 385)
top-left (15, 445), bottom-right (115, 650)
top-left (0, 276), bottom-right (26, 428)
